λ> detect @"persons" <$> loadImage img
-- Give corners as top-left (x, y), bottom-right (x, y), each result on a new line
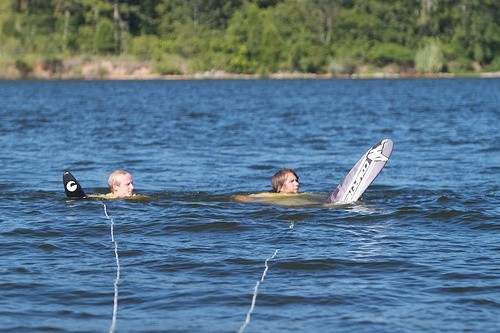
top-left (106, 168), bottom-right (136, 196)
top-left (271, 167), bottom-right (301, 194)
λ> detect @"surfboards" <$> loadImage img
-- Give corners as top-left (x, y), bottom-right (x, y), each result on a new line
top-left (62, 171), bottom-right (88, 200)
top-left (324, 139), bottom-right (394, 204)
